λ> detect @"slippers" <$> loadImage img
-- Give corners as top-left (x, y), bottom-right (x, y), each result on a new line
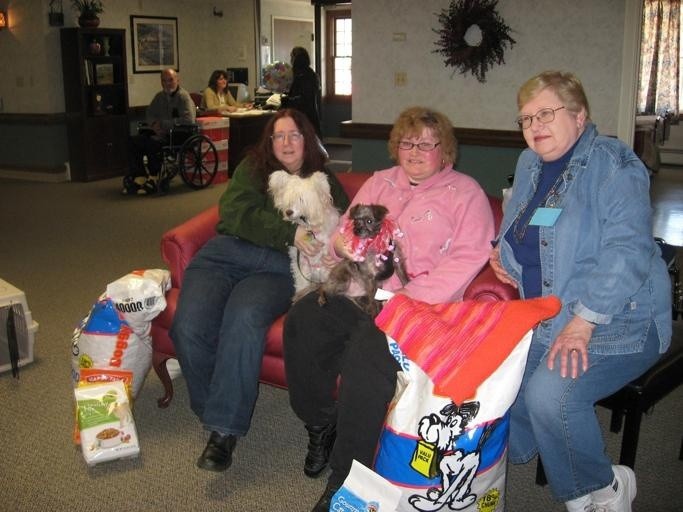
top-left (122, 179), bottom-right (159, 196)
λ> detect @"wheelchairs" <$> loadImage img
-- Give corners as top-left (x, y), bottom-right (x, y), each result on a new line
top-left (119, 118), bottom-right (218, 201)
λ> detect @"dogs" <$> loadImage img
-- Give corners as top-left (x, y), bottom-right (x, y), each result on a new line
top-left (317, 203), bottom-right (411, 319)
top-left (267, 170), bottom-right (349, 300)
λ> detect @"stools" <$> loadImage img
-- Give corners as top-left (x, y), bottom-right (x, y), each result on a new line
top-left (530, 320), bottom-right (681, 486)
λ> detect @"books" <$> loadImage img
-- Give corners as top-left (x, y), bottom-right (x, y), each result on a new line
top-left (80, 58), bottom-right (114, 86)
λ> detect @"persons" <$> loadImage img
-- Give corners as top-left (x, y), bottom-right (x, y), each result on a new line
top-left (488, 77), bottom-right (674, 512)
top-left (123, 68), bottom-right (196, 195)
top-left (277, 46), bottom-right (321, 131)
top-left (200, 70), bottom-right (254, 113)
top-left (283, 106), bottom-right (494, 512)
top-left (168, 108), bottom-right (351, 471)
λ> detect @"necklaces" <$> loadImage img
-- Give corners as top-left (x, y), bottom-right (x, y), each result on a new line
top-left (512, 160), bottom-right (572, 243)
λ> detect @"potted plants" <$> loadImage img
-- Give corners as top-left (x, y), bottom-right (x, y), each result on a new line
top-left (71, 0), bottom-right (104, 28)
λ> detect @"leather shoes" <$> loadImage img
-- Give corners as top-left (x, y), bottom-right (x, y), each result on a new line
top-left (196, 431), bottom-right (236, 472)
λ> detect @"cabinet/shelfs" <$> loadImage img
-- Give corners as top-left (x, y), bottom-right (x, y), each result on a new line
top-left (58, 27), bottom-right (131, 182)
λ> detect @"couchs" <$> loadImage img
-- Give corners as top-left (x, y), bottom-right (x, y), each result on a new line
top-left (151, 172), bottom-right (518, 410)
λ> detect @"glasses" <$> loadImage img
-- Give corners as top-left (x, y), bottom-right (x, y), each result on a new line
top-left (515, 106), bottom-right (564, 130)
top-left (269, 132), bottom-right (302, 146)
top-left (398, 141), bottom-right (440, 151)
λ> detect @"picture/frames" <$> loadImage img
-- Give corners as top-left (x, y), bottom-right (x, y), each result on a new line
top-left (129, 14), bottom-right (179, 74)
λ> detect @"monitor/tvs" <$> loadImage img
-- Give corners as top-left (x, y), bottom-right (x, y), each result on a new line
top-left (228, 83), bottom-right (246, 103)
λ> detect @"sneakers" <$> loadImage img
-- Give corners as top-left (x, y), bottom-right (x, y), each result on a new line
top-left (565, 465), bottom-right (636, 512)
top-left (304, 423), bottom-right (337, 478)
top-left (310, 472), bottom-right (349, 512)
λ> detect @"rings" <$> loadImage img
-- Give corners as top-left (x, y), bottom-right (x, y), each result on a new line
top-left (570, 348), bottom-right (578, 355)
